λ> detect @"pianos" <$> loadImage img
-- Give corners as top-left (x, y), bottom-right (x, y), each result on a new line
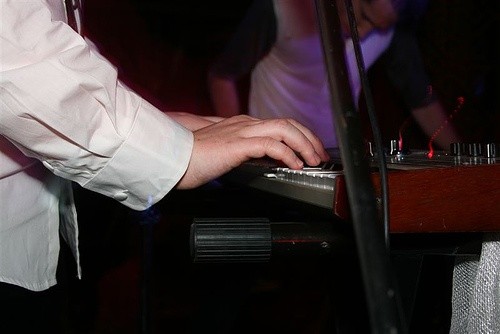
top-left (235, 140), bottom-right (500, 234)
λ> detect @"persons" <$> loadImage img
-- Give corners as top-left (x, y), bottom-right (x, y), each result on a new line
top-left (1, 0), bottom-right (331, 334)
top-left (208, 0), bottom-right (468, 160)
